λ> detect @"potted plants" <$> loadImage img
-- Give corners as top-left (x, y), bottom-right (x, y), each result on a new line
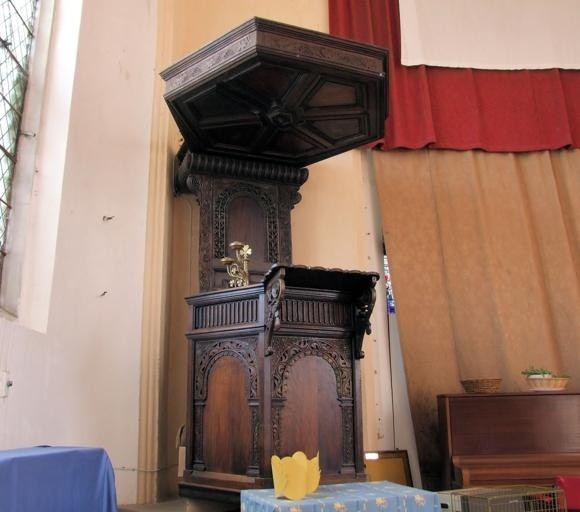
top-left (522, 367), bottom-right (552, 378)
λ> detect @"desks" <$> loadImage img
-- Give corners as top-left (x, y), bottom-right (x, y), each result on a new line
top-left (241, 481), bottom-right (442, 512)
top-left (0, 445), bottom-right (117, 512)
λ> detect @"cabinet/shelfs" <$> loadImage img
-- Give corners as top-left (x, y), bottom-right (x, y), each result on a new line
top-left (177, 263), bottom-right (380, 503)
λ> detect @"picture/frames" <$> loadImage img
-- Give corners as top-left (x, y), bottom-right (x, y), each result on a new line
top-left (365, 450), bottom-right (414, 488)
